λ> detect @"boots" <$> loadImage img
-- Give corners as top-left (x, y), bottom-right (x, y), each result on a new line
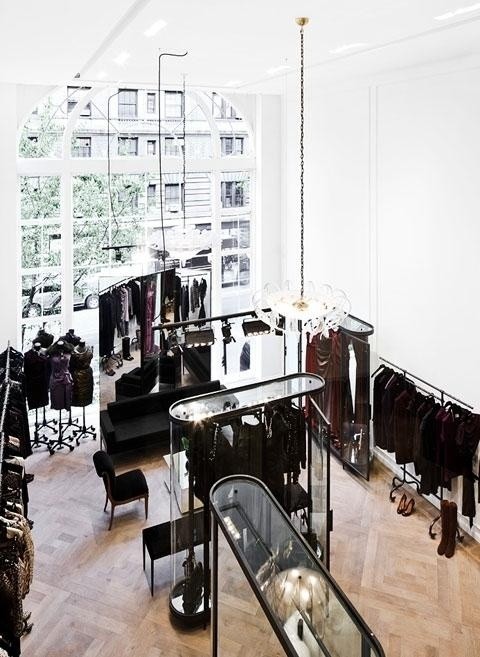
top-left (438, 500), bottom-right (457, 558)
top-left (122, 337), bottom-right (134, 361)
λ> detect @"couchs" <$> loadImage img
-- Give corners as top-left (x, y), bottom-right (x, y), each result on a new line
top-left (100, 380), bottom-right (239, 455)
top-left (115, 358), bottom-right (158, 402)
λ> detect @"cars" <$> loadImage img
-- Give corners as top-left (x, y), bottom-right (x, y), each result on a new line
top-left (22, 280), bottom-right (100, 317)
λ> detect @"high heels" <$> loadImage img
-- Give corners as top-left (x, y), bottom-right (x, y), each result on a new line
top-left (105, 365), bottom-right (115, 376)
top-left (397, 494), bottom-right (415, 516)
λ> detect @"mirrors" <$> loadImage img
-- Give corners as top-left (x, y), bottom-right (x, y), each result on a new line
top-left (98, 269), bottom-right (211, 450)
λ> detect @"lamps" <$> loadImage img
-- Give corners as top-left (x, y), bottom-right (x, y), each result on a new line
top-left (252, 17), bottom-right (351, 342)
top-left (145, 71), bottom-right (223, 268)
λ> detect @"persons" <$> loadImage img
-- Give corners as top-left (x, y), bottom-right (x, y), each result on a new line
top-left (59, 328), bottom-right (81, 346)
top-left (23, 342), bottom-right (50, 409)
top-left (68, 339), bottom-right (93, 406)
top-left (32, 329), bottom-right (55, 349)
top-left (45, 338), bottom-right (75, 410)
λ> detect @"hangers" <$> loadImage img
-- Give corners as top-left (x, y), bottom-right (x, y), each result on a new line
top-left (0, 348), bottom-right (23, 517)
top-left (371, 360), bottom-right (472, 421)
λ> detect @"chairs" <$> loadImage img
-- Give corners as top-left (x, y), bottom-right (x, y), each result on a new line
top-left (93, 450), bottom-right (148, 531)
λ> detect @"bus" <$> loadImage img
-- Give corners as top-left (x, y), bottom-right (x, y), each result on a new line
top-left (161, 250), bottom-right (250, 287)
